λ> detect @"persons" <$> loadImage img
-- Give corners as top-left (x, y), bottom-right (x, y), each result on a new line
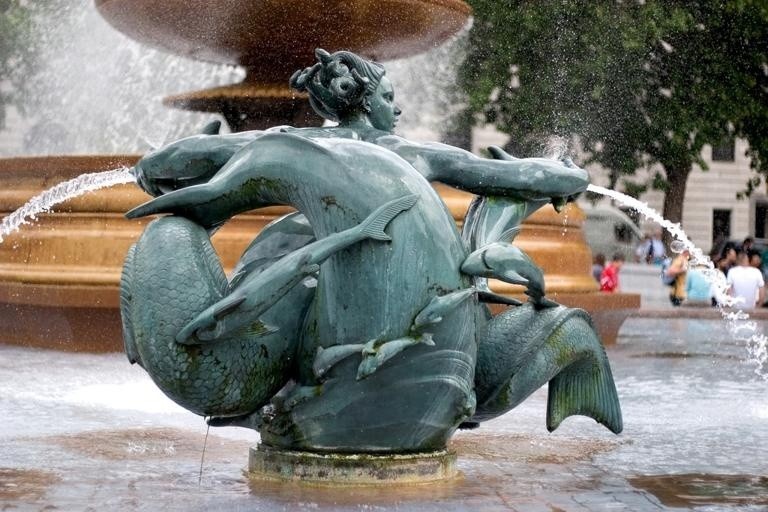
top-left (635, 226), bottom-right (768, 309)
top-left (599, 252), bottom-right (627, 292)
top-left (593, 251), bottom-right (605, 282)
top-left (118, 47), bottom-right (623, 451)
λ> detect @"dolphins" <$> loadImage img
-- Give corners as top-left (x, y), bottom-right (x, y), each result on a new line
top-left (311, 336), bottom-right (378, 380)
top-left (355, 330), bottom-right (438, 381)
top-left (410, 285), bottom-right (484, 332)
